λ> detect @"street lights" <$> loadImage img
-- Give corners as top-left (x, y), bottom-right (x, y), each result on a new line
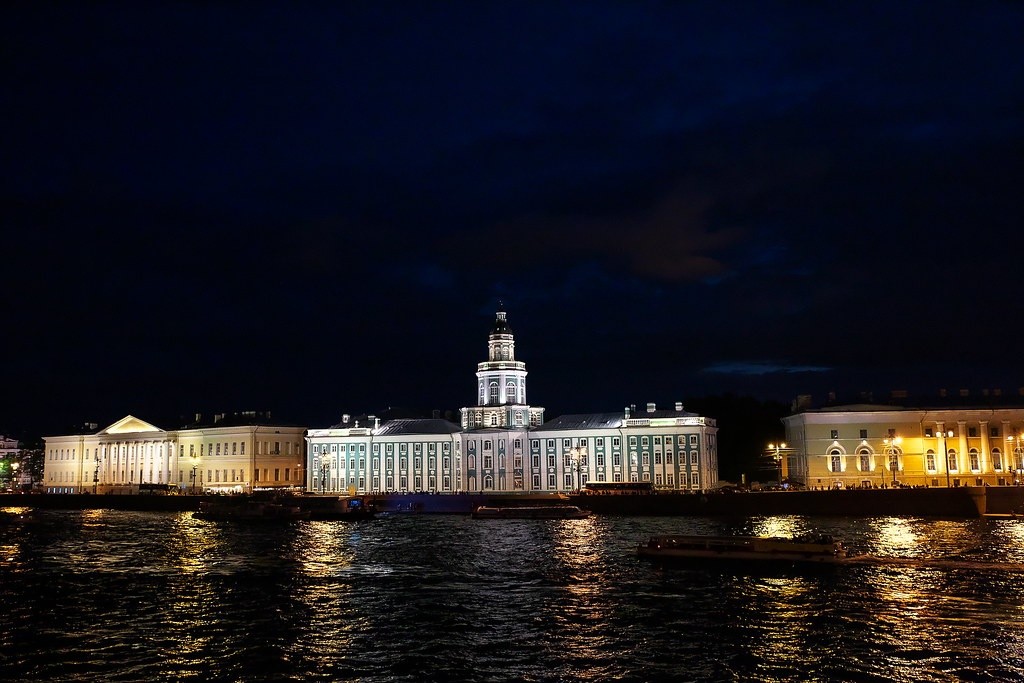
top-left (569, 446), bottom-right (586, 491)
top-left (1008, 433), bottom-right (1024, 481)
top-left (935, 429), bottom-right (954, 488)
top-left (883, 432), bottom-right (902, 484)
top-left (768, 439), bottom-right (787, 488)
top-left (320, 448), bottom-right (331, 494)
top-left (190, 452), bottom-right (201, 490)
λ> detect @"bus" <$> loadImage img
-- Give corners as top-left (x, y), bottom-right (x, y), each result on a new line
top-left (585, 481), bottom-right (657, 497)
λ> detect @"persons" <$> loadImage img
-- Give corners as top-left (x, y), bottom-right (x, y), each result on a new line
top-left (104, 483), bottom-right (932, 496)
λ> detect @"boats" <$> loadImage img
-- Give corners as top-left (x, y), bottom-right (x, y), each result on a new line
top-left (470, 505), bottom-right (593, 519)
top-left (199, 497), bottom-right (312, 520)
top-left (344, 495), bottom-right (420, 514)
top-left (636, 524), bottom-right (856, 567)
